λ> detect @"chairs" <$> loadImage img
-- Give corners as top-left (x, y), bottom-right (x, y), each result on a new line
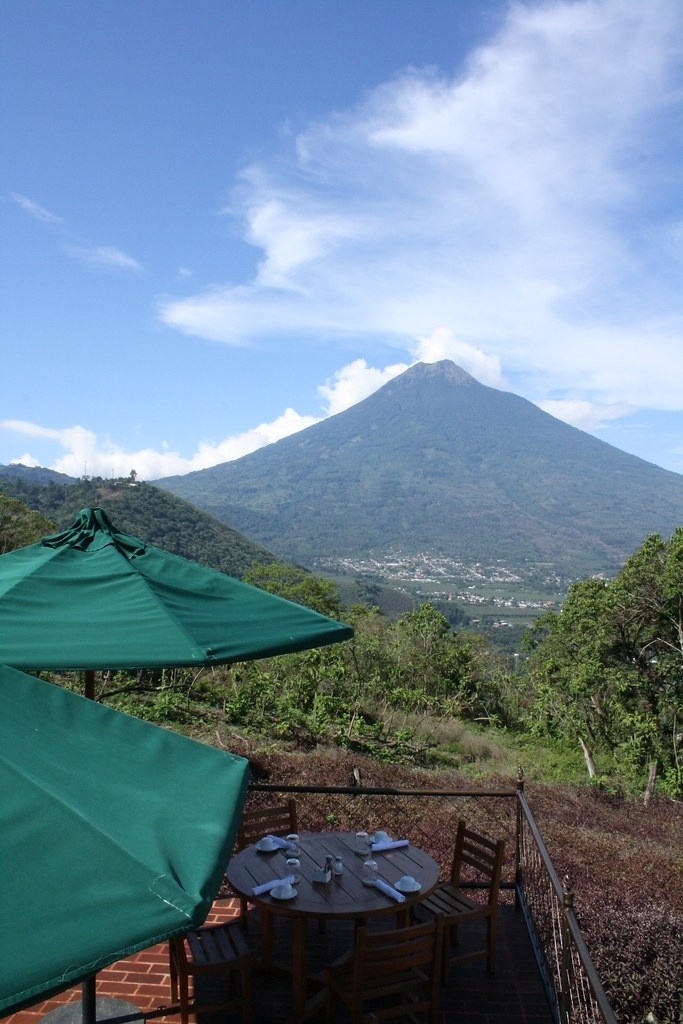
top-left (169, 921), bottom-right (252, 1024)
top-left (411, 819), bottom-right (506, 985)
top-left (325, 913), bottom-right (442, 1024)
top-left (238, 798), bottom-right (298, 931)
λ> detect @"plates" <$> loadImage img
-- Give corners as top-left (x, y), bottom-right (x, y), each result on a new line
top-left (270, 888), bottom-right (297, 900)
top-left (255, 843), bottom-right (279, 851)
top-left (370, 836), bottom-right (393, 843)
top-left (394, 881), bottom-right (421, 892)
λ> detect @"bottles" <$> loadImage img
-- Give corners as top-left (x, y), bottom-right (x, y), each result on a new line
top-left (334, 856), bottom-right (343, 874)
top-left (324, 855), bottom-right (333, 874)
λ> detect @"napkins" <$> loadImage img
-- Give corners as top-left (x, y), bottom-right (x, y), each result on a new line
top-left (252, 875), bottom-right (295, 895)
top-left (372, 840), bottom-right (409, 851)
top-left (269, 835), bottom-right (296, 850)
top-left (374, 879), bottom-right (406, 903)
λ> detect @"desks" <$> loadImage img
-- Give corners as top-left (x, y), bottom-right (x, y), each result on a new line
top-left (226, 832), bottom-right (440, 1024)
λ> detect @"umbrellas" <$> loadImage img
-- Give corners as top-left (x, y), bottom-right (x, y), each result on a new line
top-left (0, 662), bottom-right (249, 1024)
top-left (0, 507), bottom-right (355, 700)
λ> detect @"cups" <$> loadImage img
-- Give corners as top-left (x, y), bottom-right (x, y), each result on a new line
top-left (400, 876), bottom-right (419, 890)
top-left (374, 831), bottom-right (388, 843)
top-left (286, 834), bottom-right (300, 857)
top-left (286, 858), bottom-right (300, 883)
top-left (256, 837), bottom-right (273, 849)
top-left (355, 832), bottom-right (369, 855)
top-left (362, 861), bottom-right (379, 886)
top-left (276, 882), bottom-right (292, 898)
